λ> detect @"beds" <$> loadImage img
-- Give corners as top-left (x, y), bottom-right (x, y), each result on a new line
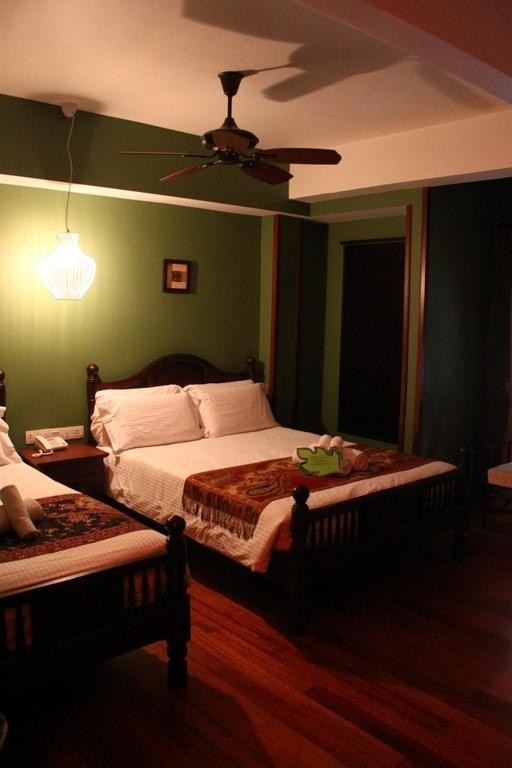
top-left (73, 353), bottom-right (471, 636)
top-left (0, 372), bottom-right (192, 696)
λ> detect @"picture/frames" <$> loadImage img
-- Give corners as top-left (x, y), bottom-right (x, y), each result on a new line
top-left (163, 260), bottom-right (191, 294)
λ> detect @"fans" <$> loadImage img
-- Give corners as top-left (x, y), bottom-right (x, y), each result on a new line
top-left (116, 70), bottom-right (341, 185)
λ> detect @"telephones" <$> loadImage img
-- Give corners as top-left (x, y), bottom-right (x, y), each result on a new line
top-left (34, 435), bottom-right (69, 453)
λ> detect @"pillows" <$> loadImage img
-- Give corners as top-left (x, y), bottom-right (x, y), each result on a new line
top-left (89, 378), bottom-right (277, 455)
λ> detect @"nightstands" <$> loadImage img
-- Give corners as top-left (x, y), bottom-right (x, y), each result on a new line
top-left (19, 442), bottom-right (109, 499)
